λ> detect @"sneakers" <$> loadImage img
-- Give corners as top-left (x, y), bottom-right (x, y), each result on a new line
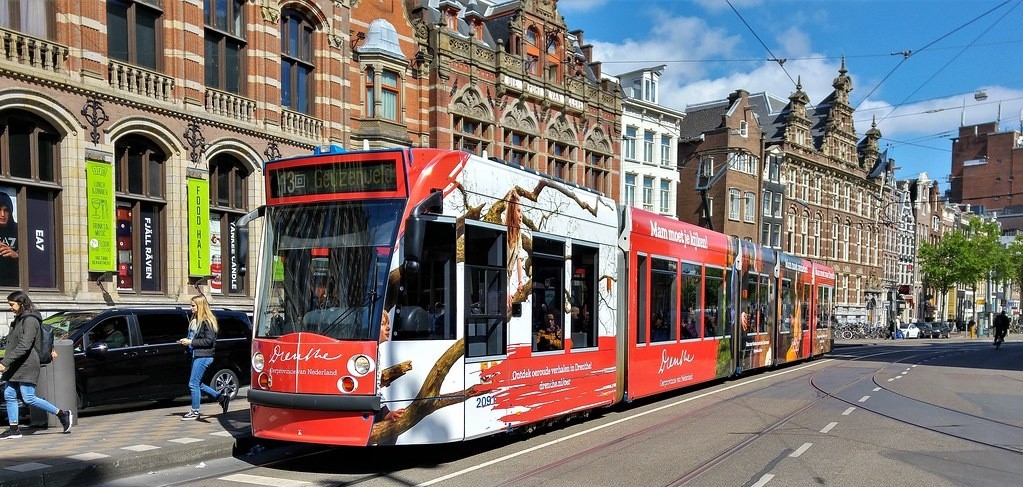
top-left (219, 395), bottom-right (230, 415)
top-left (181, 410), bottom-right (200, 420)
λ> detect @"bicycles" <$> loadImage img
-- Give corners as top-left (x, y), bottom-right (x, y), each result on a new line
top-left (996, 335), bottom-right (1004, 349)
top-left (833, 319), bottom-right (892, 340)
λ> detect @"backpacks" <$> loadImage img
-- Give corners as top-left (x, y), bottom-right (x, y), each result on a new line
top-left (22, 316), bottom-right (54, 365)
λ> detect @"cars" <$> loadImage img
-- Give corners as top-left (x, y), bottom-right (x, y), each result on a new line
top-left (913, 323), bottom-right (934, 339)
top-left (899, 323), bottom-right (922, 339)
top-left (932, 322), bottom-right (951, 339)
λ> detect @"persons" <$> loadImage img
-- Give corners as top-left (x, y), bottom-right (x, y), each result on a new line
top-left (740, 246), bottom-right (749, 366)
top-left (432, 302), bottom-right (444, 337)
top-left (268, 308), bottom-right (284, 335)
top-left (0, 291), bottom-right (73, 439)
top-left (888, 321), bottom-right (894, 336)
top-left (99, 319), bottom-right (125, 348)
top-left (992, 310), bottom-right (1009, 345)
top-left (310, 271), bottom-right (341, 334)
top-left (179, 295), bottom-right (230, 420)
top-left (506, 194), bottom-right (524, 311)
top-left (376, 310), bottom-right (405, 447)
top-left (0, 192), bottom-right (19, 286)
top-left (651, 306), bottom-right (718, 342)
top-left (571, 304), bottom-right (589, 333)
top-left (536, 312), bottom-right (562, 351)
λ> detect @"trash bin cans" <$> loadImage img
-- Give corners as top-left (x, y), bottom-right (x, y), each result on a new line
top-left (30, 339), bottom-right (79, 427)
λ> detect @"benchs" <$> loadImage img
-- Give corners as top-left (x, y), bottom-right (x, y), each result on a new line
top-left (532, 332), bottom-right (587, 351)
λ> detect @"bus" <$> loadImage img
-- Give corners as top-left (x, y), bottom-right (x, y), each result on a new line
top-left (234, 148), bottom-right (838, 447)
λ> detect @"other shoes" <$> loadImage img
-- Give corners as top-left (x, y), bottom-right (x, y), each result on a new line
top-left (58, 410), bottom-right (71, 433)
top-left (993, 343), bottom-right (997, 345)
top-left (0, 429), bottom-right (23, 440)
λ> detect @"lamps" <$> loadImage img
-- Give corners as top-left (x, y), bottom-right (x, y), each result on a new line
top-left (526, 57), bottom-right (538, 71)
top-left (195, 275), bottom-right (217, 288)
top-left (410, 51), bottom-right (425, 68)
top-left (975, 89), bottom-right (988, 100)
top-left (567, 69), bottom-right (582, 86)
top-left (352, 31), bottom-right (366, 48)
top-left (96, 271), bottom-right (120, 285)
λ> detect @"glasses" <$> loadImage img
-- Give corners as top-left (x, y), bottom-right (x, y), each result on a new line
top-left (191, 305), bottom-right (196, 307)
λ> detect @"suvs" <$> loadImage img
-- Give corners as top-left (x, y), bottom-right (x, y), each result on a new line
top-left (0, 304), bottom-right (252, 419)
